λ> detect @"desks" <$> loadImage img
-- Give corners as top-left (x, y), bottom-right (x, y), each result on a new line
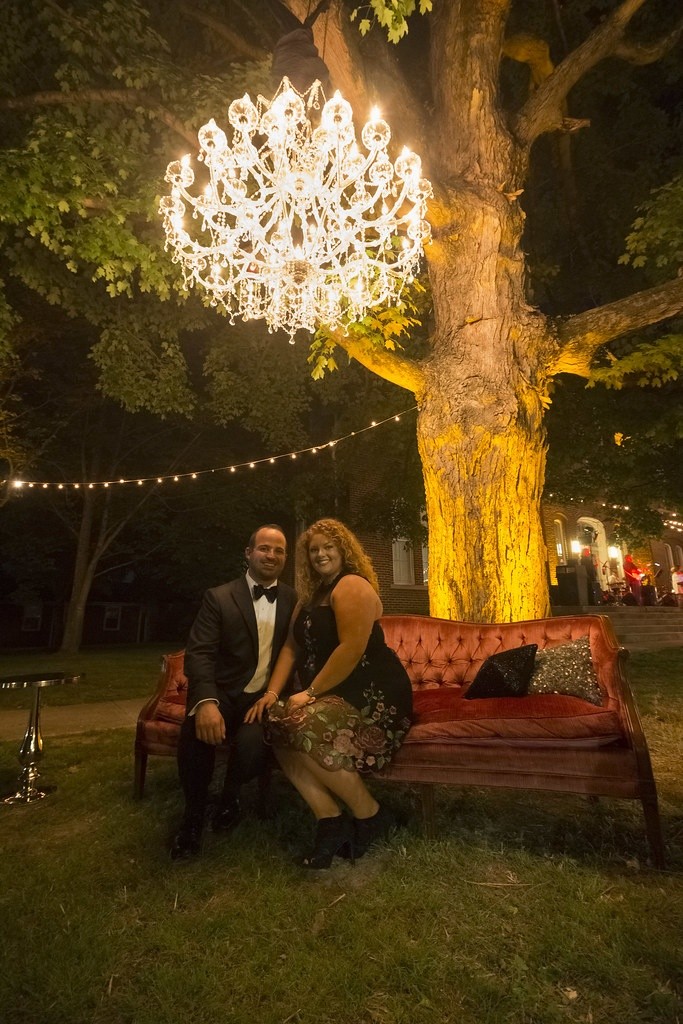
top-left (0, 672), bottom-right (86, 807)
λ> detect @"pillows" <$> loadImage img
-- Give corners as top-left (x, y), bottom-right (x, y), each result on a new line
top-left (528, 635), bottom-right (603, 707)
top-left (461, 643), bottom-right (538, 701)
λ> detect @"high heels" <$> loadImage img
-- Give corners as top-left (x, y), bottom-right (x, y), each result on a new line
top-left (292, 815), bottom-right (353, 869)
top-left (352, 800), bottom-right (395, 859)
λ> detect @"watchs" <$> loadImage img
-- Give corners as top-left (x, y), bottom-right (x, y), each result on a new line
top-left (306, 686), bottom-right (321, 699)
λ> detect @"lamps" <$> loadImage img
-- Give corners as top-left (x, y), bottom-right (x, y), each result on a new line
top-left (158, 75), bottom-right (435, 346)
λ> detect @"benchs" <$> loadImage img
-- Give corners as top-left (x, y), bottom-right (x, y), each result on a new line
top-left (133, 614), bottom-right (666, 875)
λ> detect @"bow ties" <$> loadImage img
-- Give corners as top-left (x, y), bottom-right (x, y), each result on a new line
top-left (253, 585), bottom-right (277, 603)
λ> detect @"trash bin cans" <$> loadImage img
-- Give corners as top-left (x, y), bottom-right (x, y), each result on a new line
top-left (556, 565), bottom-right (588, 605)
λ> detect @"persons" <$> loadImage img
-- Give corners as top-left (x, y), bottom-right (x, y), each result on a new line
top-left (579, 548), bottom-right (683, 607)
top-left (261, 518), bottom-right (416, 870)
top-left (169, 522), bottom-right (301, 869)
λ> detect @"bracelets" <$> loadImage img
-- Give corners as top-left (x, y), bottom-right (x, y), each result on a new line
top-left (263, 690), bottom-right (279, 701)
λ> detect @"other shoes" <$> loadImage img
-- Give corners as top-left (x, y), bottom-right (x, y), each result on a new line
top-left (213, 779), bottom-right (242, 831)
top-left (172, 816), bottom-right (202, 859)
